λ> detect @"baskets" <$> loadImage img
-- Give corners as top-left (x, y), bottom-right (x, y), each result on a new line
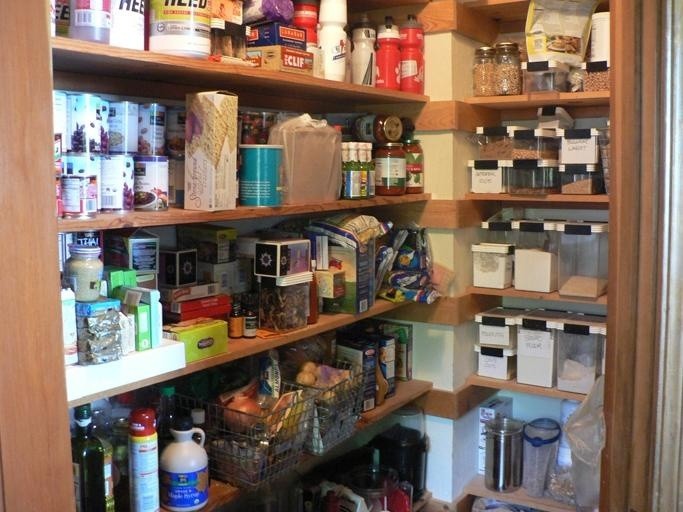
top-left (149, 372), bottom-right (324, 495)
top-left (276, 356), bottom-right (366, 459)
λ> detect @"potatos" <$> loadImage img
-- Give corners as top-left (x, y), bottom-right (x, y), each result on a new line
top-left (295, 361), bottom-right (357, 403)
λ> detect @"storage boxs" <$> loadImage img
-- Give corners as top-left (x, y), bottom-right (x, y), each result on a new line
top-left (513, 308), bottom-right (574, 389)
top-left (473, 304), bottom-right (530, 350)
top-left (511, 214), bottom-right (568, 297)
top-left (556, 216), bottom-right (608, 304)
top-left (469, 217), bottom-right (515, 290)
top-left (554, 310), bottom-right (609, 394)
top-left (463, 123), bottom-right (609, 197)
top-left (472, 342), bottom-right (518, 381)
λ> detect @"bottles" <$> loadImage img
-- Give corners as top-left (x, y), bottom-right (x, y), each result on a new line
top-left (319, 0), bottom-right (351, 81)
top-left (350, 13), bottom-right (378, 86)
top-left (244, 300), bottom-right (259, 339)
top-left (373, 14), bottom-right (401, 90)
top-left (62, 243), bottom-right (103, 302)
top-left (228, 301), bottom-right (244, 339)
top-left (70, 403), bottom-right (105, 512)
top-left (472, 39), bottom-right (521, 95)
top-left (293, 0), bottom-right (319, 79)
top-left (556, 398), bottom-right (587, 469)
top-left (298, 475), bottom-right (340, 511)
top-left (338, 140), bottom-right (375, 200)
top-left (398, 12), bottom-right (426, 95)
top-left (340, 464), bottom-right (413, 511)
top-left (89, 385), bottom-right (210, 512)
top-left (482, 415), bottom-right (522, 493)
top-left (522, 61), bottom-right (610, 92)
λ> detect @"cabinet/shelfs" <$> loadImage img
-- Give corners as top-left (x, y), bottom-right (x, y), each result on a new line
top-left (461, 1), bottom-right (663, 511)
top-left (4, 1), bottom-right (432, 511)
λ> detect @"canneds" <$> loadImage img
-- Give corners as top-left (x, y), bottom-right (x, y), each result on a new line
top-left (237, 110), bottom-right (274, 168)
top-left (374, 142), bottom-right (407, 196)
top-left (351, 112), bottom-right (403, 144)
top-left (53, 89), bottom-right (186, 219)
top-left (403, 139), bottom-right (425, 194)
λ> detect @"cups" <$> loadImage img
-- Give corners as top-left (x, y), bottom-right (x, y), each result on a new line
top-left (522, 416), bottom-right (561, 501)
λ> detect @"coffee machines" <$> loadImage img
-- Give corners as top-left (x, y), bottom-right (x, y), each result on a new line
top-left (366, 405), bottom-right (426, 502)
